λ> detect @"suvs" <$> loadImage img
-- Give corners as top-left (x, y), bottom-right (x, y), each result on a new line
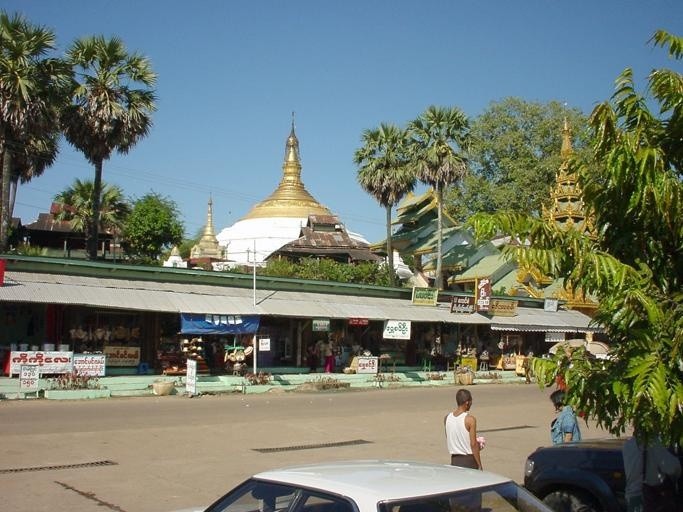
top-left (523, 432), bottom-right (682, 511)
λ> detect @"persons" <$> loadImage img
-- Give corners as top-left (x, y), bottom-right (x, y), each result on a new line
top-left (443, 390), bottom-right (483, 470)
top-left (308, 339), bottom-right (334, 372)
top-left (623, 436), bottom-right (683, 512)
top-left (521, 355), bottom-right (531, 384)
top-left (550, 390), bottom-right (580, 447)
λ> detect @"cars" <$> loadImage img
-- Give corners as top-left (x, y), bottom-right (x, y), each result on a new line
top-left (189, 461), bottom-right (552, 512)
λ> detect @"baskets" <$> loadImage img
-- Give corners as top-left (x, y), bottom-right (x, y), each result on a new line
top-left (152, 381), bottom-right (175, 395)
top-left (454, 372), bottom-right (474, 384)
